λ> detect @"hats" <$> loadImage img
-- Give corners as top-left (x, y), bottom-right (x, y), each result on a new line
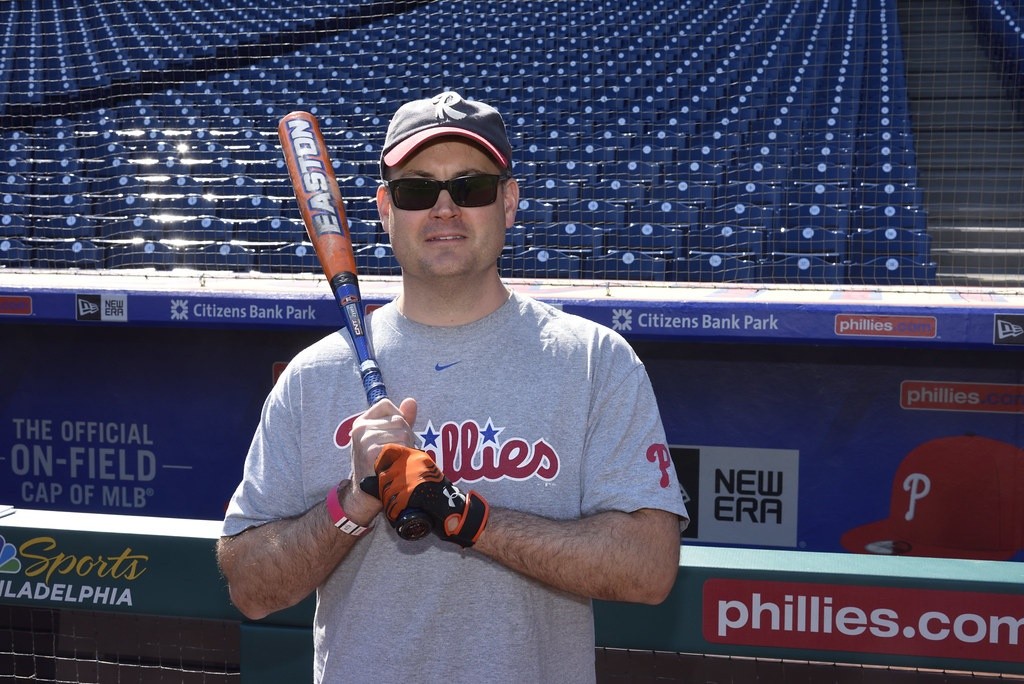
top-left (380, 91), bottom-right (513, 181)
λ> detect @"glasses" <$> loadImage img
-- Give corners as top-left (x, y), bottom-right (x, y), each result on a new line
top-left (384, 174), bottom-right (509, 212)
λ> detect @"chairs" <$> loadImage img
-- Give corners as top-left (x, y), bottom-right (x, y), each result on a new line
top-left (0, 0), bottom-right (1024, 287)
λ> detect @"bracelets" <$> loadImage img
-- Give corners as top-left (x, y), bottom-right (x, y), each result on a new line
top-left (328, 479), bottom-right (375, 537)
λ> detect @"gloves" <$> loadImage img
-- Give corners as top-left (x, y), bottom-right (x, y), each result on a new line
top-left (360, 443), bottom-right (489, 548)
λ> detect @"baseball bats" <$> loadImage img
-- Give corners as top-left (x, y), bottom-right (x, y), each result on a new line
top-left (277, 110), bottom-right (430, 544)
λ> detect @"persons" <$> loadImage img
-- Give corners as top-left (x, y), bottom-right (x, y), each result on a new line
top-left (219, 91), bottom-right (690, 684)
